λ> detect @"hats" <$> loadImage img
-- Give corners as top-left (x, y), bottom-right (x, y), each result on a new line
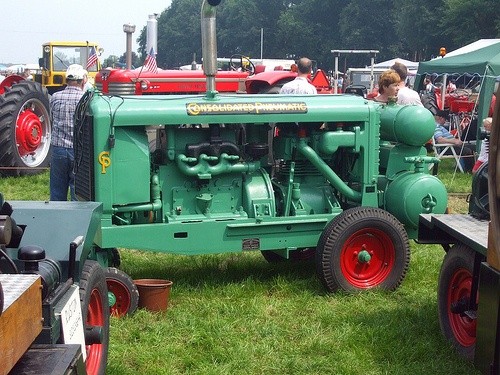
top-left (437, 110), bottom-right (449, 120)
top-left (65, 64), bottom-right (84, 80)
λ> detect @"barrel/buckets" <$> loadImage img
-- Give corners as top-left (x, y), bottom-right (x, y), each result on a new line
top-left (133, 278), bottom-right (175, 313)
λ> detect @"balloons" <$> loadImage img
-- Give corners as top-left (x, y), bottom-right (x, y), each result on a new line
top-left (338, 80), bottom-right (341, 87)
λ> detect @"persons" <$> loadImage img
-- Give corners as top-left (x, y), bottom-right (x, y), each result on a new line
top-left (49, 63), bottom-right (88, 203)
top-left (278, 57), bottom-right (318, 95)
top-left (331, 76), bottom-right (335, 83)
top-left (374, 71), bottom-right (400, 103)
top-left (341, 72), bottom-right (348, 84)
top-left (434, 110), bottom-right (475, 172)
top-left (447, 79), bottom-right (456, 91)
top-left (390, 61), bottom-right (422, 104)
top-left (425, 80), bottom-right (435, 92)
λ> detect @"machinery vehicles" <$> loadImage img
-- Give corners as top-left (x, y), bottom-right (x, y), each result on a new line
top-left (73, 0), bottom-right (447, 319)
top-left (0, 192), bottom-right (110, 375)
top-left (413, 82), bottom-right (500, 375)
top-left (0, 12), bottom-right (415, 177)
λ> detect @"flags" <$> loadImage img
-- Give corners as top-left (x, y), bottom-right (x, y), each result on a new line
top-left (144, 48), bottom-right (159, 76)
top-left (86, 49), bottom-right (98, 68)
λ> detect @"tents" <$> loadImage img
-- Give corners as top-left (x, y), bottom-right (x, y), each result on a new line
top-left (413, 39), bottom-right (500, 153)
